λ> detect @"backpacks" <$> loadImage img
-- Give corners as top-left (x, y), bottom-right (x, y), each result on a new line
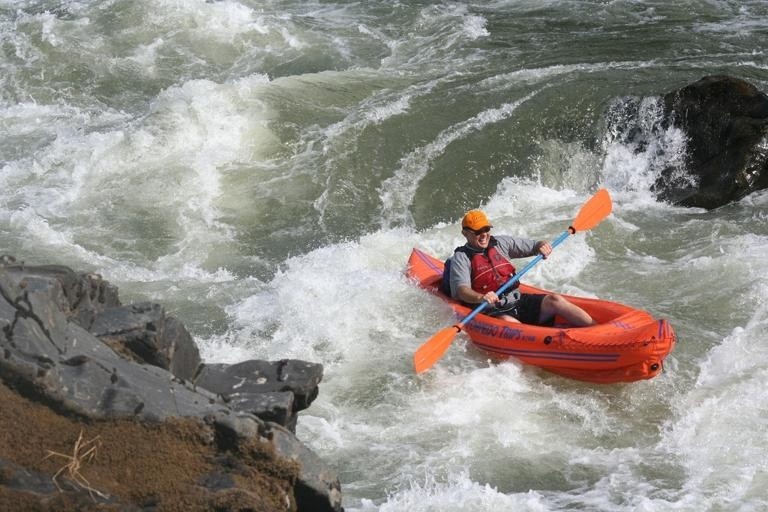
top-left (443, 246), bottom-right (473, 298)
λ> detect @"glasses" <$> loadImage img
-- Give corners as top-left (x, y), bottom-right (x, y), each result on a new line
top-left (464, 227), bottom-right (490, 236)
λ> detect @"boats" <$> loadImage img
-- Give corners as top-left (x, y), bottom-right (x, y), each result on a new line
top-left (402, 246), bottom-right (675, 384)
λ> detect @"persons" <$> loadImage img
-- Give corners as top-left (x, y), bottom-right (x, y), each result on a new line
top-left (449, 209), bottom-right (600, 327)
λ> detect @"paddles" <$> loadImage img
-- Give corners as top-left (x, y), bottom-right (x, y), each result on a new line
top-left (414, 190), bottom-right (612, 374)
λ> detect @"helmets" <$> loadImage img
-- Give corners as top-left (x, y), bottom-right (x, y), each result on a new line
top-left (462, 210), bottom-right (493, 230)
